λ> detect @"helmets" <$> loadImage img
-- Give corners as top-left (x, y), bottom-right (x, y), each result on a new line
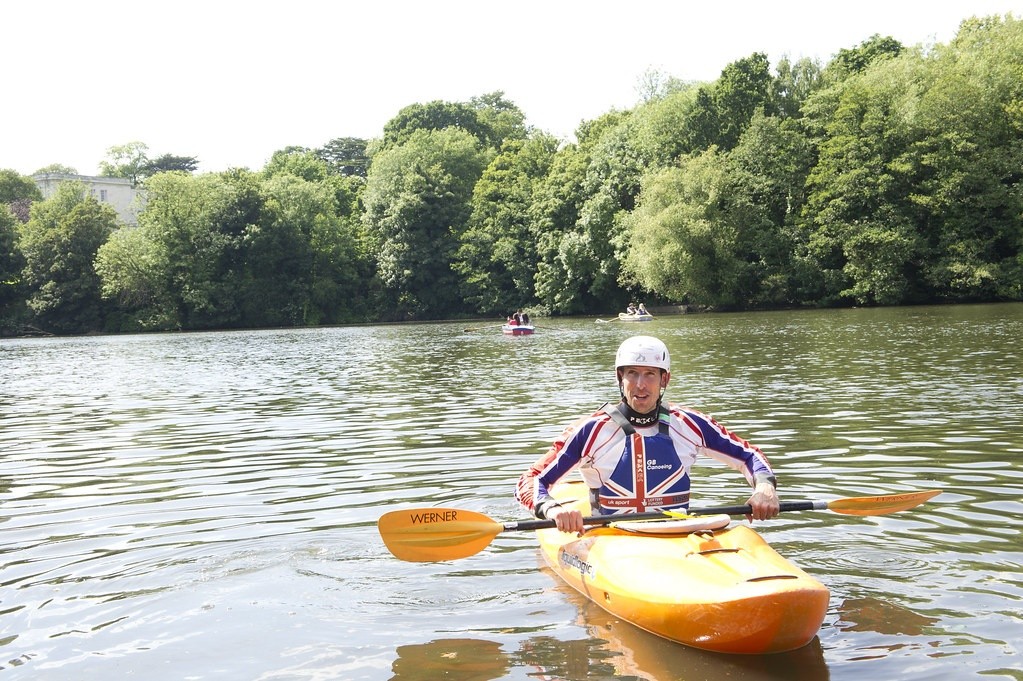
top-left (615, 335), bottom-right (670, 382)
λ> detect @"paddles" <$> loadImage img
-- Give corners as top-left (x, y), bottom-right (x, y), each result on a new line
top-left (376, 488), bottom-right (943, 563)
top-left (463, 324), bottom-right (566, 333)
top-left (607, 312), bottom-right (632, 322)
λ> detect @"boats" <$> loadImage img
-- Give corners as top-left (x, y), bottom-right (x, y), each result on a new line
top-left (502, 324), bottom-right (535, 335)
top-left (535, 476), bottom-right (830, 654)
top-left (618, 313), bottom-right (653, 321)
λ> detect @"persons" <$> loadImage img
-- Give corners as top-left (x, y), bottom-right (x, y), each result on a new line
top-left (513, 308), bottom-right (525, 323)
top-left (500, 336), bottom-right (783, 536)
top-left (637, 302), bottom-right (647, 316)
top-left (626, 303), bottom-right (640, 314)
top-left (520, 313), bottom-right (532, 327)
top-left (505, 313), bottom-right (521, 326)
